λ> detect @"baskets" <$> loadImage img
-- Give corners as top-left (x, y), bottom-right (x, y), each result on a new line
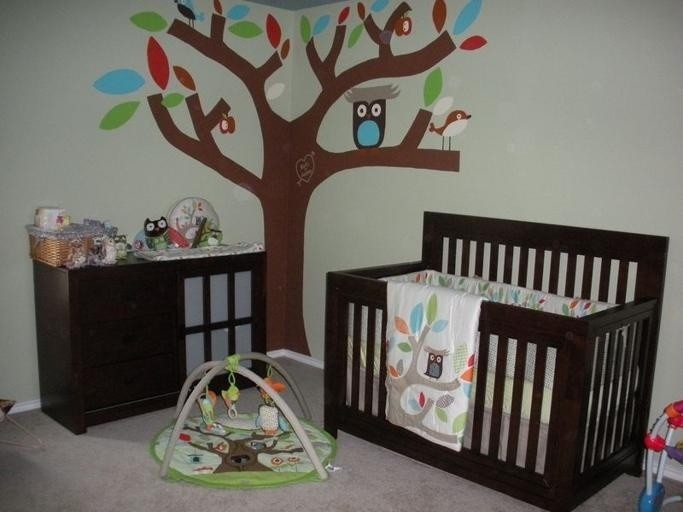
top-left (26, 225), bottom-right (104, 267)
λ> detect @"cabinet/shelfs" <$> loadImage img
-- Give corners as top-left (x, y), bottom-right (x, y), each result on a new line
top-left (30, 241), bottom-right (268, 436)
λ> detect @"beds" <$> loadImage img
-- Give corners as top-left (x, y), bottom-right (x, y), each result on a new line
top-left (321, 210), bottom-right (671, 511)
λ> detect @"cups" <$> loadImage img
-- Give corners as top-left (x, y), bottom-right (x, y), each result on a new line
top-left (34, 208), bottom-right (66, 230)
